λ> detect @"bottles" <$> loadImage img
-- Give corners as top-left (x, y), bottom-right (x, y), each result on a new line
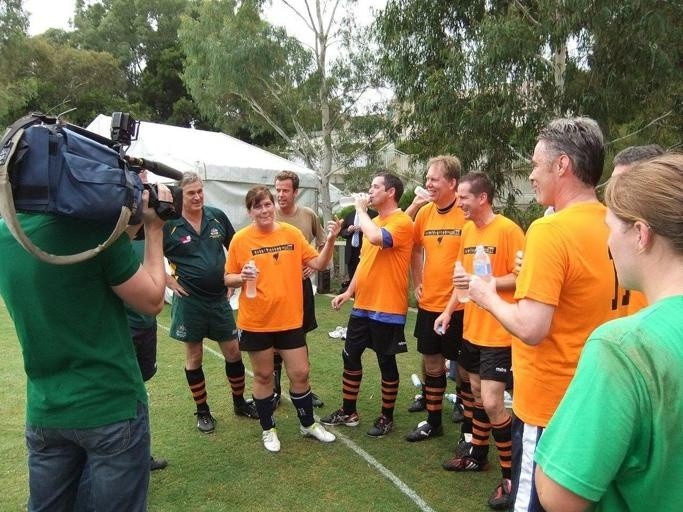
top-left (414, 186), bottom-right (432, 204)
top-left (445, 393), bottom-right (456, 405)
top-left (245, 259), bottom-right (257, 299)
top-left (472, 244), bottom-right (492, 311)
top-left (338, 193), bottom-right (371, 207)
top-left (503, 390), bottom-right (512, 409)
top-left (454, 260), bottom-right (470, 304)
top-left (411, 373), bottom-right (422, 388)
top-left (545, 205), bottom-right (555, 215)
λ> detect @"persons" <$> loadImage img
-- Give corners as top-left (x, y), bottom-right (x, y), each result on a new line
top-left (611, 144), bottom-right (664, 178)
top-left (404, 156), bottom-right (525, 507)
top-left (320, 172), bottom-right (415, 439)
top-left (0, 181), bottom-right (174, 512)
top-left (223, 186), bottom-right (345, 452)
top-left (469, 117), bottom-right (629, 511)
top-left (535, 151), bottom-right (682, 510)
top-left (125, 169), bottom-right (168, 472)
top-left (273, 171), bottom-right (327, 408)
top-left (340, 192), bottom-right (379, 298)
top-left (163, 171), bottom-right (260, 434)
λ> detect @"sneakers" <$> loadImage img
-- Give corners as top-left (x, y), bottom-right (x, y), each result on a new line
top-left (443, 433), bottom-right (488, 472)
top-left (262, 427), bottom-right (280, 452)
top-left (408, 398), bottom-right (426, 411)
top-left (488, 478), bottom-right (512, 509)
top-left (452, 403), bottom-right (464, 421)
top-left (320, 407), bottom-right (359, 426)
top-left (312, 394), bottom-right (323, 407)
top-left (301, 423), bottom-right (335, 442)
top-left (197, 410), bottom-right (215, 433)
top-left (234, 393), bottom-right (281, 419)
top-left (407, 420), bottom-right (443, 441)
top-left (367, 413), bottom-right (393, 437)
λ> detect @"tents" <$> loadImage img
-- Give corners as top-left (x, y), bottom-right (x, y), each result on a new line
top-left (85, 113), bottom-right (321, 311)
